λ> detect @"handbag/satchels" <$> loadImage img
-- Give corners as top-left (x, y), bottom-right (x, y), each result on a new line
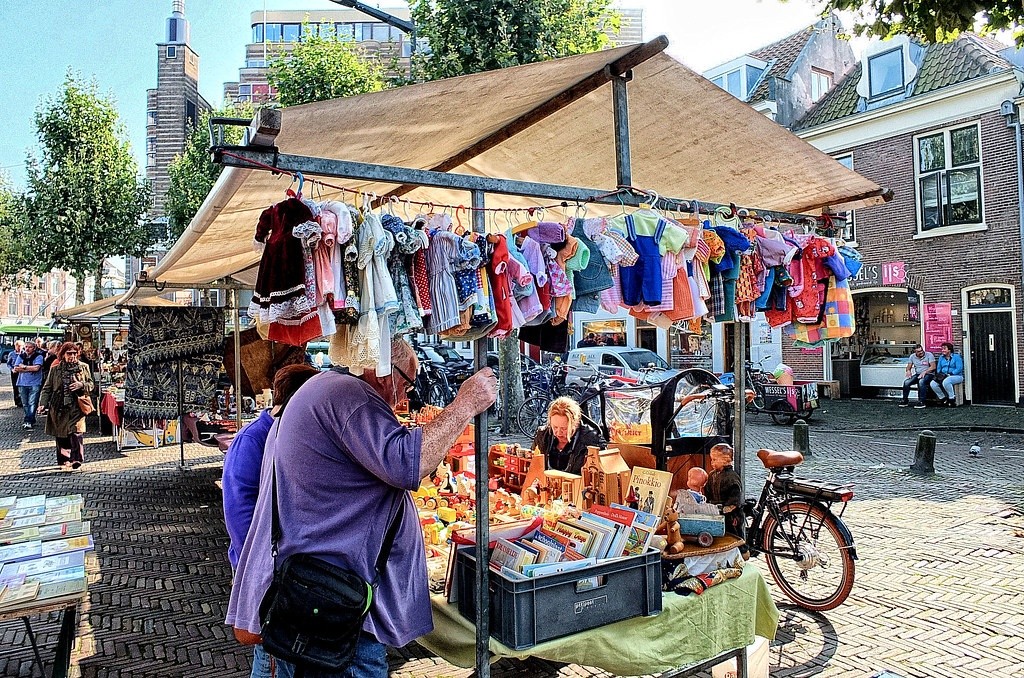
top-left (935, 372), bottom-right (947, 384)
top-left (77, 395), bottom-right (95, 415)
top-left (258, 553), bottom-right (373, 670)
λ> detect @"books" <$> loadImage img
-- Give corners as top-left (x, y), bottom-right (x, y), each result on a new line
top-left (0, 493), bottom-right (94, 613)
top-left (490, 465), bottom-right (673, 581)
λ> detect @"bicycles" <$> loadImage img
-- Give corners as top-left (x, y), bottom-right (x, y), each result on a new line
top-left (410, 357), bottom-right (611, 441)
top-left (605, 390), bottom-right (858, 612)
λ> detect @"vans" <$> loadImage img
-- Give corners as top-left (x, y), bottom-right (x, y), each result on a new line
top-left (566, 346), bottom-right (678, 396)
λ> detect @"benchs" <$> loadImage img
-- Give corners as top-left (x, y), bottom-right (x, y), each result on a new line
top-left (793, 378), bottom-right (840, 400)
top-left (917, 381), bottom-right (963, 406)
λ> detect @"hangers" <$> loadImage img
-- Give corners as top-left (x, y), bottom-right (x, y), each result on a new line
top-left (277, 172), bottom-right (847, 249)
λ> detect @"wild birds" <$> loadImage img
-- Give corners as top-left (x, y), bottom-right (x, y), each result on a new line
top-left (969, 441), bottom-right (980, 456)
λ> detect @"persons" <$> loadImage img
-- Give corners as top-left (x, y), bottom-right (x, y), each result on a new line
top-left (222, 333), bottom-right (499, 678)
top-left (674, 444), bottom-right (752, 560)
top-left (898, 344), bottom-right (936, 409)
top-left (930, 343), bottom-right (964, 407)
top-left (530, 397), bottom-right (607, 477)
top-left (7, 335), bottom-right (96, 470)
top-left (679, 348), bottom-right (695, 368)
top-left (577, 333), bottom-right (626, 348)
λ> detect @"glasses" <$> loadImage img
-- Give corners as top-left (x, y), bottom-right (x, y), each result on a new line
top-left (391, 361), bottom-right (416, 394)
top-left (65, 351), bottom-right (78, 356)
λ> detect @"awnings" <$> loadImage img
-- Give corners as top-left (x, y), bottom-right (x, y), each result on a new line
top-left (149, 42), bottom-right (892, 299)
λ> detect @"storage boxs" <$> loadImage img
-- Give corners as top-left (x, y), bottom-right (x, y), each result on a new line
top-left (453, 545), bottom-right (663, 651)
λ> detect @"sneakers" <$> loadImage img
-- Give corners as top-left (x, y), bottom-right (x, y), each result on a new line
top-left (899, 400), bottom-right (908, 407)
top-left (914, 402), bottom-right (926, 408)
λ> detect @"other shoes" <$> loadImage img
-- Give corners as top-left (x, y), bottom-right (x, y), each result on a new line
top-left (60, 460), bottom-right (71, 470)
top-left (71, 460), bottom-right (81, 469)
top-left (16, 403), bottom-right (24, 409)
top-left (22, 422), bottom-right (33, 430)
top-left (938, 397), bottom-right (957, 407)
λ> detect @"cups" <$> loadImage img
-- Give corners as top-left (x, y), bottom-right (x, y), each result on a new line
top-left (256, 394), bottom-right (265, 408)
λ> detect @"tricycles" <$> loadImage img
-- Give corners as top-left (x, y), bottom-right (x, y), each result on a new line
top-left (730, 356), bottom-right (818, 425)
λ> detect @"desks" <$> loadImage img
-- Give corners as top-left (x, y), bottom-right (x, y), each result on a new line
top-left (180, 412), bottom-right (748, 678)
top-left (93, 372), bottom-right (126, 441)
top-left (0, 497), bottom-right (88, 678)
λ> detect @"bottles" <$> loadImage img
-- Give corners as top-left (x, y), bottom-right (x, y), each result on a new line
top-left (262, 388), bottom-right (272, 407)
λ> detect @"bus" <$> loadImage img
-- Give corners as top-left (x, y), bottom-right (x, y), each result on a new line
top-left (0, 325), bottom-right (65, 363)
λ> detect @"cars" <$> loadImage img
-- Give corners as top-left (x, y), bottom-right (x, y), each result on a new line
top-left (306, 342), bottom-right (329, 353)
top-left (415, 343), bottom-right (473, 383)
top-left (470, 350), bottom-right (539, 372)
